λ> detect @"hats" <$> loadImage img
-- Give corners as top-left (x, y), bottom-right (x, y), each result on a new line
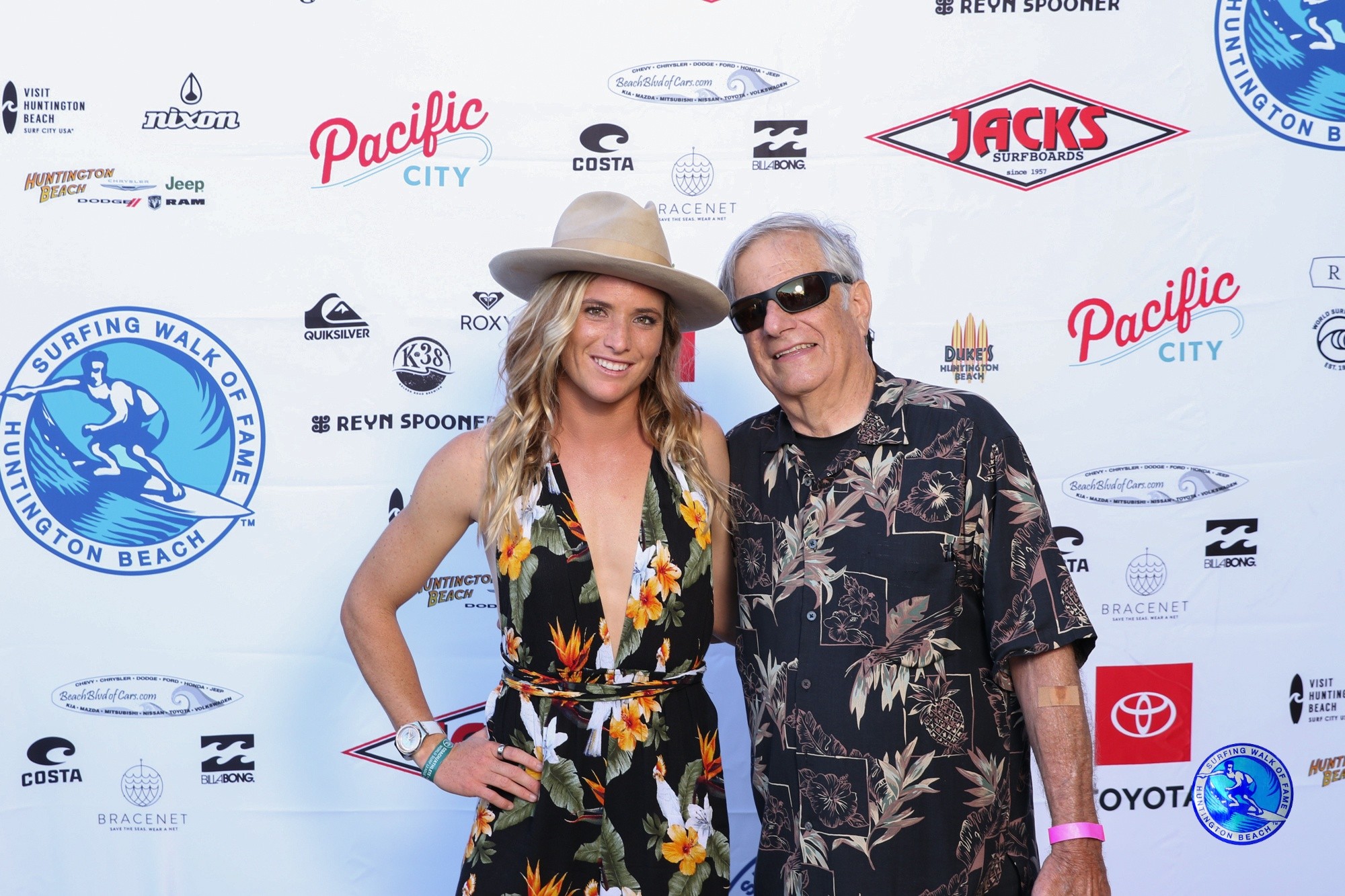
top-left (489, 191), bottom-right (730, 332)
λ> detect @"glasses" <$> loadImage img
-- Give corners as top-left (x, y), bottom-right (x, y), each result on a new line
top-left (729, 270), bottom-right (853, 334)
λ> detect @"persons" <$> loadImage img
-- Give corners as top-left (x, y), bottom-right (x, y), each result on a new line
top-left (339, 190), bottom-right (735, 896)
top-left (725, 213), bottom-right (1112, 896)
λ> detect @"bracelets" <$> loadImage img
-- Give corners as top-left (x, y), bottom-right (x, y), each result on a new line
top-left (1048, 822), bottom-right (1105, 845)
top-left (422, 738), bottom-right (455, 782)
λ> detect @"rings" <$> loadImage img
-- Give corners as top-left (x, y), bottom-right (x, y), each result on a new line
top-left (497, 744), bottom-right (508, 760)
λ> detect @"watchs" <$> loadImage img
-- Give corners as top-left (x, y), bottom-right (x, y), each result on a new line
top-left (394, 721), bottom-right (447, 761)
